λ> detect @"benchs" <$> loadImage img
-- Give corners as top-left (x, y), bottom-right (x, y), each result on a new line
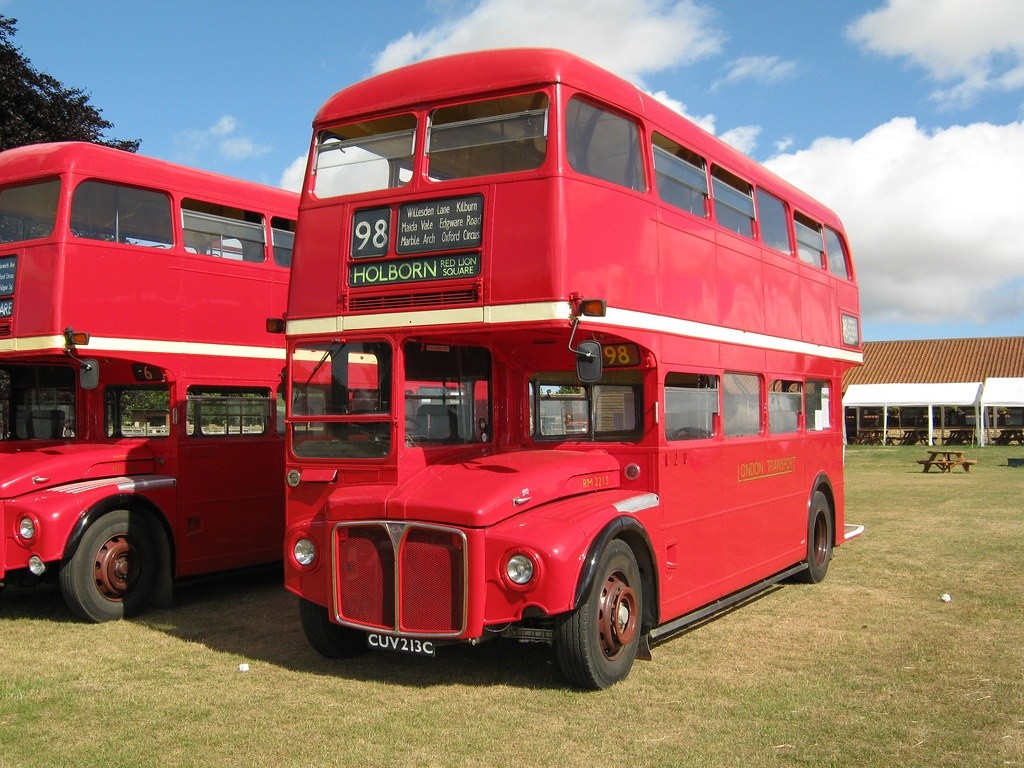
top-left (848, 436), bottom-right (1024, 473)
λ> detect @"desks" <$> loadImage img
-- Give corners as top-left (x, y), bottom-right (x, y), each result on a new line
top-left (923, 451), bottom-right (972, 473)
top-left (995, 430), bottom-right (1024, 446)
top-left (899, 430), bottom-right (936, 445)
top-left (945, 430), bottom-right (976, 445)
top-left (854, 430), bottom-right (894, 445)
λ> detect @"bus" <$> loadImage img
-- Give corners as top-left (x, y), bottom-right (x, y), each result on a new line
top-left (282, 46), bottom-right (864, 690)
top-left (0, 141), bottom-right (491, 623)
